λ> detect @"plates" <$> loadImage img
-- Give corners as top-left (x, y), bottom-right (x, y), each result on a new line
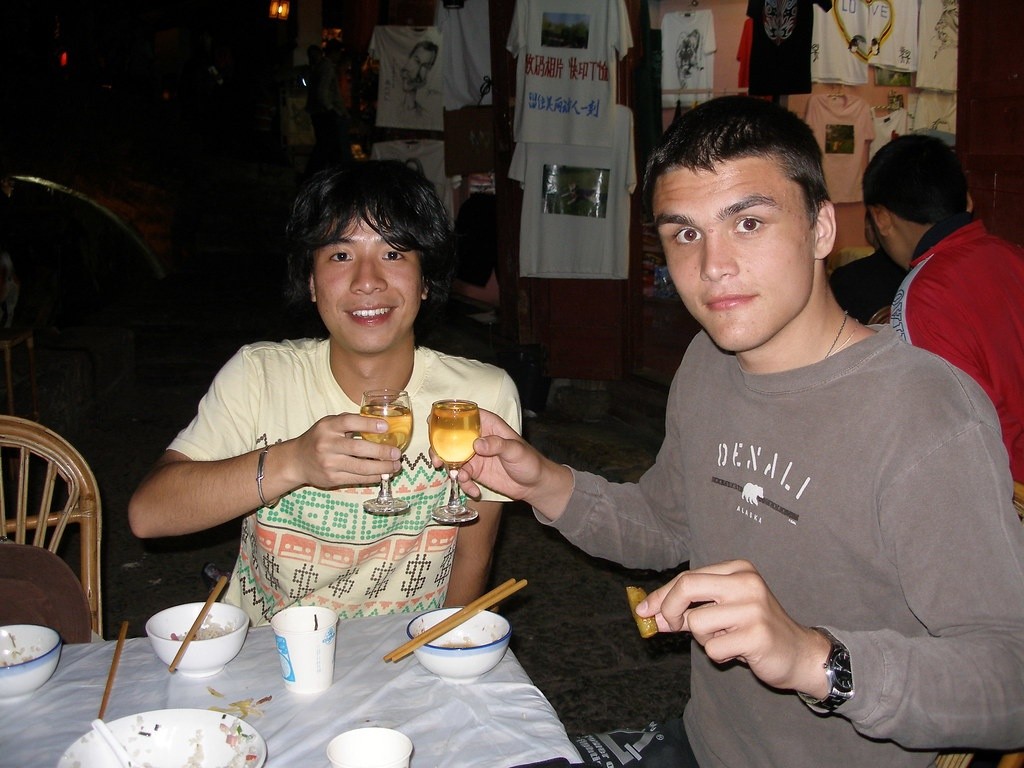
top-left (56, 708), bottom-right (267, 768)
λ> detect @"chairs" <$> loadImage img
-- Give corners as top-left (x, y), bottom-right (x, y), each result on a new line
top-left (0, 252), bottom-right (42, 424)
top-left (0, 413), bottom-right (102, 640)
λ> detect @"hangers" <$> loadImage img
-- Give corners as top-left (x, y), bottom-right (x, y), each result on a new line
top-left (827, 83), bottom-right (845, 97)
top-left (872, 90), bottom-right (914, 121)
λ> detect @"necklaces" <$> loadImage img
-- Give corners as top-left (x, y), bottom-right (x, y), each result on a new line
top-left (825, 309), bottom-right (859, 358)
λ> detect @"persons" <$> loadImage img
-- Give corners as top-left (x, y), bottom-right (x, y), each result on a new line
top-left (122, 158), bottom-right (525, 631)
top-left (829, 210), bottom-right (908, 327)
top-left (860, 134), bottom-right (1024, 488)
top-left (426, 94), bottom-right (1024, 767)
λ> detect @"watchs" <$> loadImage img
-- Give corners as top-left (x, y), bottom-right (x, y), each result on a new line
top-left (795, 626), bottom-right (854, 712)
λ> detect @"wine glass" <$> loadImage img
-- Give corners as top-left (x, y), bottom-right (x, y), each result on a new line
top-left (358, 388), bottom-right (413, 513)
top-left (429, 398), bottom-right (482, 523)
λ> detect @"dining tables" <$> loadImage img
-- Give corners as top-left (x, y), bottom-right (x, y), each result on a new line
top-left (0, 607), bottom-right (587, 767)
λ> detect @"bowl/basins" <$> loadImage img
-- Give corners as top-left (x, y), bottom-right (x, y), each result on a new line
top-left (146, 601), bottom-right (250, 677)
top-left (0, 624), bottom-right (61, 702)
top-left (406, 607), bottom-right (512, 686)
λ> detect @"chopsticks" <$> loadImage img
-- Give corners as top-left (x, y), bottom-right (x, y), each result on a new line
top-left (97, 575), bottom-right (228, 719)
top-left (382, 577), bottom-right (528, 662)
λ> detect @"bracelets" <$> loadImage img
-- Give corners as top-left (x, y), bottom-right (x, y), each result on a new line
top-left (256, 444), bottom-right (280, 506)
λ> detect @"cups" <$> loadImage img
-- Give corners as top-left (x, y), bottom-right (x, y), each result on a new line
top-left (326, 727), bottom-right (413, 768)
top-left (270, 605), bottom-right (339, 695)
